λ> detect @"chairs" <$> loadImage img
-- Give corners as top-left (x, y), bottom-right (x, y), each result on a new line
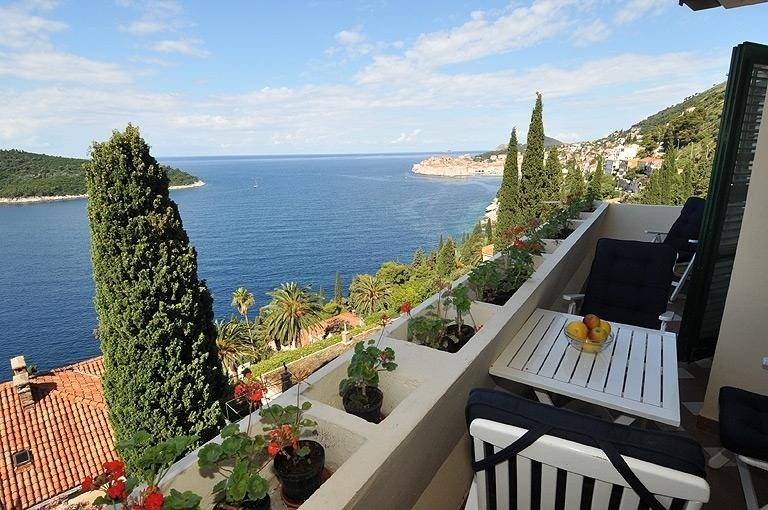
top-left (644, 196), bottom-right (706, 305)
top-left (561, 236), bottom-right (684, 331)
top-left (463, 385), bottom-right (711, 509)
top-left (706, 356), bottom-right (767, 510)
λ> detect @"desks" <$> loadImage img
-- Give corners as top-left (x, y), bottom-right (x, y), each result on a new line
top-left (487, 307), bottom-right (684, 428)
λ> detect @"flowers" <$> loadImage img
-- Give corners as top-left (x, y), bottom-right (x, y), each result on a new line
top-left (339, 315), bottom-right (399, 408)
top-left (469, 186), bottom-right (596, 306)
top-left (401, 279), bottom-right (472, 348)
top-left (197, 381), bottom-right (299, 504)
top-left (79, 434), bottom-right (203, 510)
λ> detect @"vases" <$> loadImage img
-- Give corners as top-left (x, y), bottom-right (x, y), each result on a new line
top-left (342, 386), bottom-right (383, 423)
top-left (216, 493), bottom-right (271, 510)
top-left (419, 324), bottom-right (476, 353)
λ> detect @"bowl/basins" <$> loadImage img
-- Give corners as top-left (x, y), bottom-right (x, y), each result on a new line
top-left (563, 327), bottom-right (615, 354)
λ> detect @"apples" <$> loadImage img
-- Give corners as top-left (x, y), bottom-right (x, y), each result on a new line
top-left (567, 314), bottom-right (611, 353)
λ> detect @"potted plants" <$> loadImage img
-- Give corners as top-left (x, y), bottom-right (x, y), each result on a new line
top-left (258, 402), bottom-right (326, 510)
